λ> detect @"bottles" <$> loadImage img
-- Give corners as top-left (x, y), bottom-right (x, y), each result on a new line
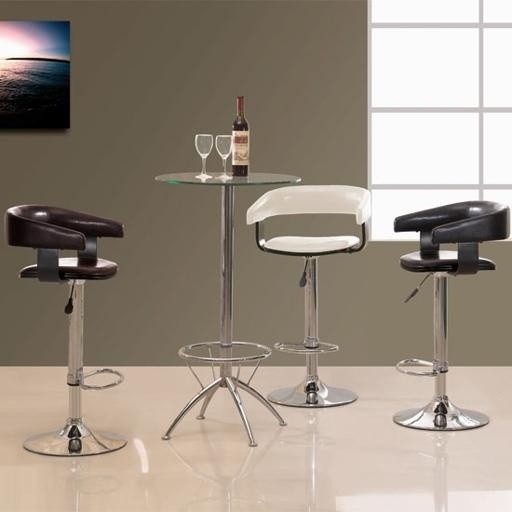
top-left (231, 96), bottom-right (250, 176)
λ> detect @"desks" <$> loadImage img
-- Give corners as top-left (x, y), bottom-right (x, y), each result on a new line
top-left (155, 172), bottom-right (302, 447)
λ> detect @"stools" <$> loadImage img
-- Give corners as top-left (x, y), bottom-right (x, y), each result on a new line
top-left (244, 185), bottom-right (373, 410)
top-left (6, 203), bottom-right (127, 456)
top-left (393, 201), bottom-right (512, 434)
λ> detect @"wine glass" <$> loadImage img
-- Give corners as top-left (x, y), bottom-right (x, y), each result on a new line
top-left (215, 135), bottom-right (233, 180)
top-left (195, 134), bottom-right (213, 178)
